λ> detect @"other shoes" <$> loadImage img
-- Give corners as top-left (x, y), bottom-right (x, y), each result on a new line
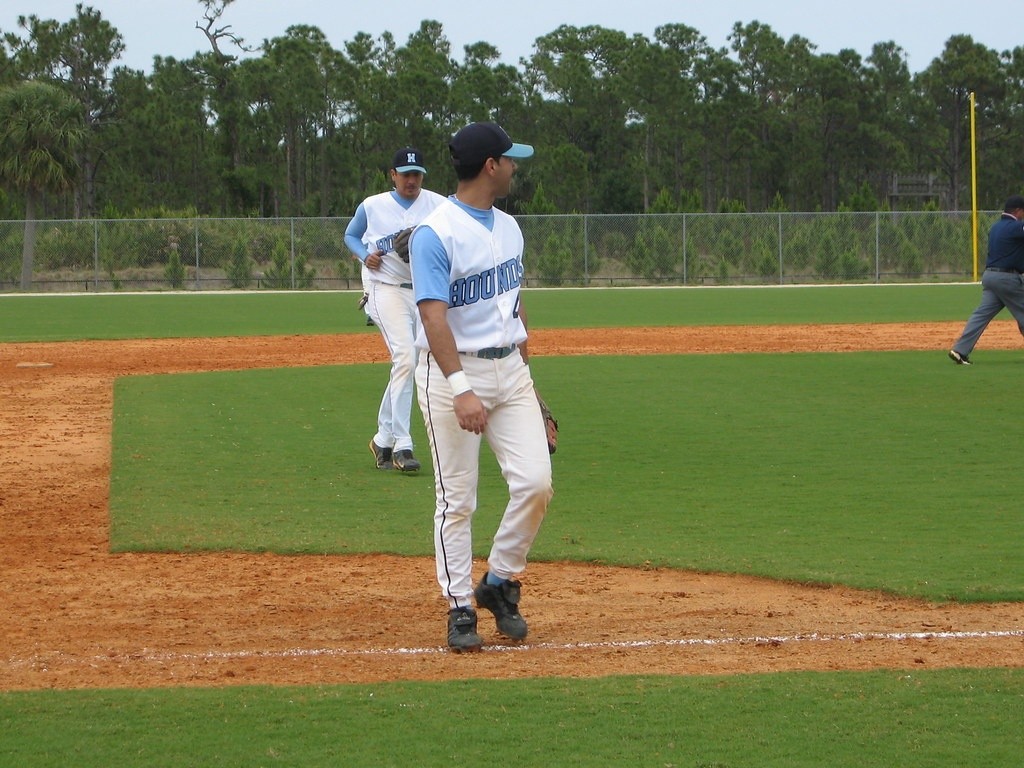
top-left (367, 316), bottom-right (374, 326)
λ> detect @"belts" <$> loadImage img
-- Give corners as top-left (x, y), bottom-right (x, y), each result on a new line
top-left (457, 342), bottom-right (519, 360)
top-left (986, 267), bottom-right (1016, 274)
top-left (381, 281), bottom-right (413, 291)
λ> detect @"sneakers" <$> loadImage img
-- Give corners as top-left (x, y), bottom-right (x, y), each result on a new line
top-left (948, 350), bottom-right (971, 366)
top-left (446, 606), bottom-right (483, 653)
top-left (472, 571), bottom-right (529, 640)
top-left (368, 439), bottom-right (421, 472)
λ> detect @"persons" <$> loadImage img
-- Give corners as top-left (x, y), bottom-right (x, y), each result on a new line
top-left (358, 258), bottom-right (375, 325)
top-left (344, 148), bottom-right (447, 473)
top-left (948, 195), bottom-right (1024, 365)
top-left (408, 122), bottom-right (552, 651)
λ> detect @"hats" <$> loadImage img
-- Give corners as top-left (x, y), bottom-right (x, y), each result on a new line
top-left (1006, 196), bottom-right (1024, 208)
top-left (447, 121), bottom-right (534, 160)
top-left (391, 146), bottom-right (426, 175)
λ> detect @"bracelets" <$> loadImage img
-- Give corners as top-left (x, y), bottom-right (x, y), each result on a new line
top-left (446, 370), bottom-right (472, 397)
